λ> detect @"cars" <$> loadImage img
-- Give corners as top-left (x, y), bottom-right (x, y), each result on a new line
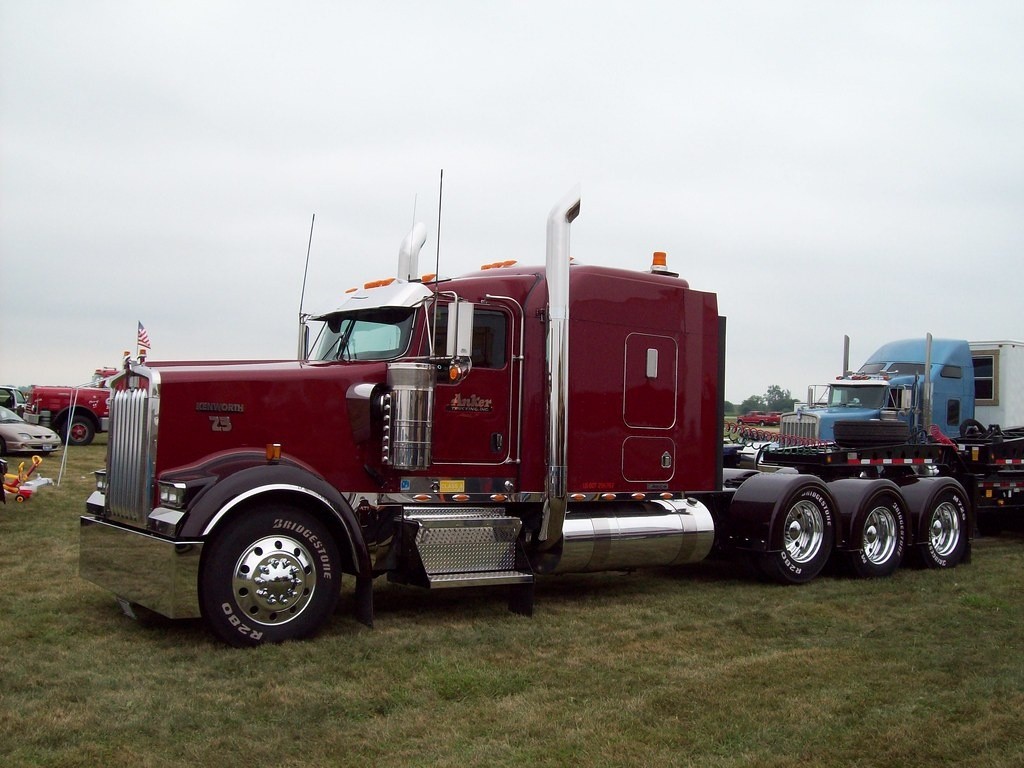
top-left (736, 411), bottom-right (772, 426)
top-left (0, 386), bottom-right (28, 418)
top-left (767, 411), bottom-right (783, 424)
top-left (1, 407), bottom-right (61, 457)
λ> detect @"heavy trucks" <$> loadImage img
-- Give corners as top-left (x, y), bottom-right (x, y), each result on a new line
top-left (779, 332), bottom-right (1024, 455)
top-left (80, 200), bottom-right (973, 650)
top-left (22, 365), bottom-right (123, 448)
